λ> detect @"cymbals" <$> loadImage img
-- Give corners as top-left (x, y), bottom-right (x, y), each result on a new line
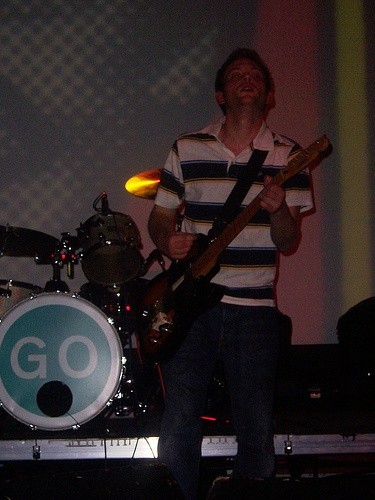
top-left (0, 224), bottom-right (61, 259)
top-left (124, 165), bottom-right (164, 205)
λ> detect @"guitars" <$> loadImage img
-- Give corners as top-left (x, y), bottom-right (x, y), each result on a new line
top-left (137, 132), bottom-right (337, 359)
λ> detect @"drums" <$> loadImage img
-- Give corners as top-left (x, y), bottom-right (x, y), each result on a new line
top-left (0, 288), bottom-right (129, 433)
top-left (75, 210), bottom-right (145, 288)
top-left (0, 279), bottom-right (43, 320)
top-left (76, 275), bottom-right (158, 341)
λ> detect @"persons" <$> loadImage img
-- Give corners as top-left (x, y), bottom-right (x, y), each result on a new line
top-left (146, 46), bottom-right (313, 500)
